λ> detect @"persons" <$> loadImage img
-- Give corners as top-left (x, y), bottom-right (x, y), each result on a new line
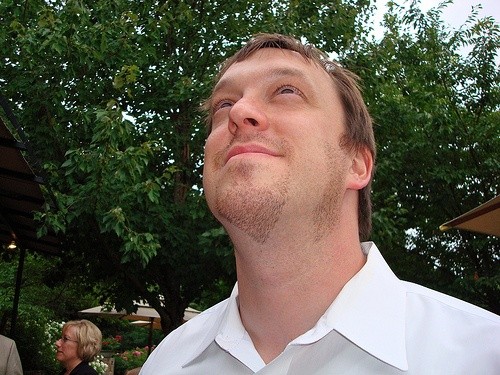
top-left (54, 320), bottom-right (103, 375)
top-left (140, 34), bottom-right (500, 375)
top-left (0, 335), bottom-right (24, 375)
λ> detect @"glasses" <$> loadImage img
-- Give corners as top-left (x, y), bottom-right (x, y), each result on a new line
top-left (60, 336), bottom-right (78, 343)
